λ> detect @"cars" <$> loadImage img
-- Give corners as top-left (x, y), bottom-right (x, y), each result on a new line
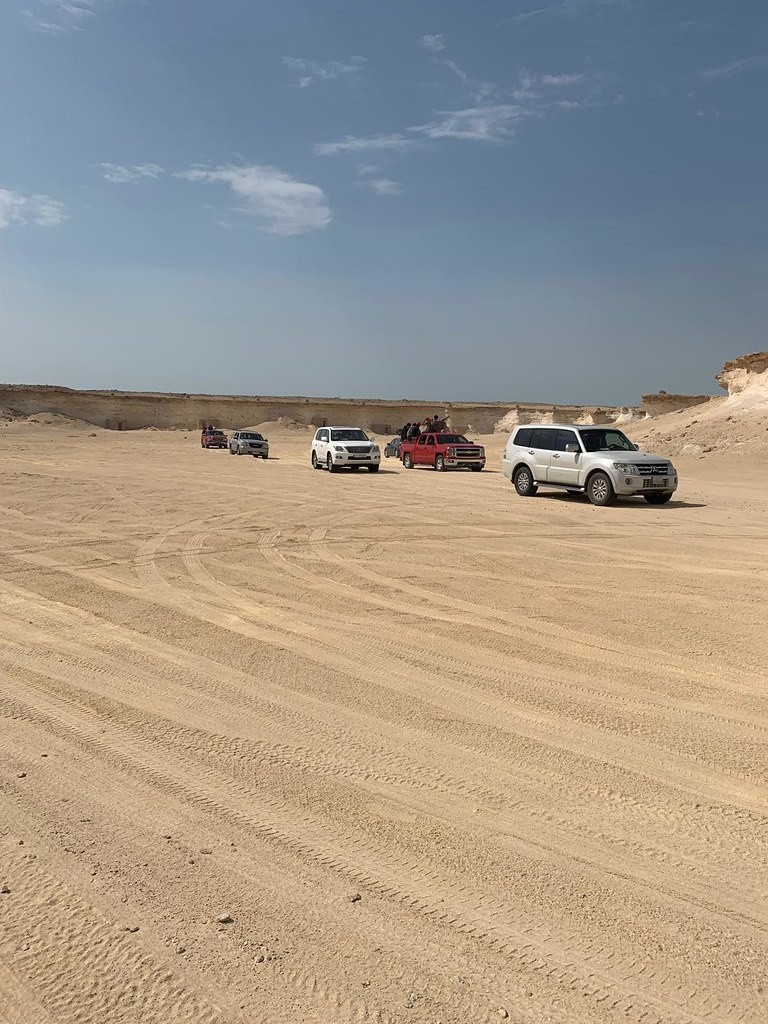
top-left (384, 437), bottom-right (402, 458)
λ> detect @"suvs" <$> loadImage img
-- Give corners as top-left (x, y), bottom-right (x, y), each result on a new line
top-left (228, 430), bottom-right (269, 460)
top-left (502, 422), bottom-right (679, 507)
top-left (310, 425), bottom-right (381, 473)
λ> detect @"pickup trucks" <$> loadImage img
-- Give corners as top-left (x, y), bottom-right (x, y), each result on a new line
top-left (200, 429), bottom-right (228, 448)
top-left (399, 431), bottom-right (486, 472)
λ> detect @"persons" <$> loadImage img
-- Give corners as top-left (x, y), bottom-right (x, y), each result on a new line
top-left (401, 415), bottom-right (450, 440)
top-left (202, 425), bottom-right (215, 436)
